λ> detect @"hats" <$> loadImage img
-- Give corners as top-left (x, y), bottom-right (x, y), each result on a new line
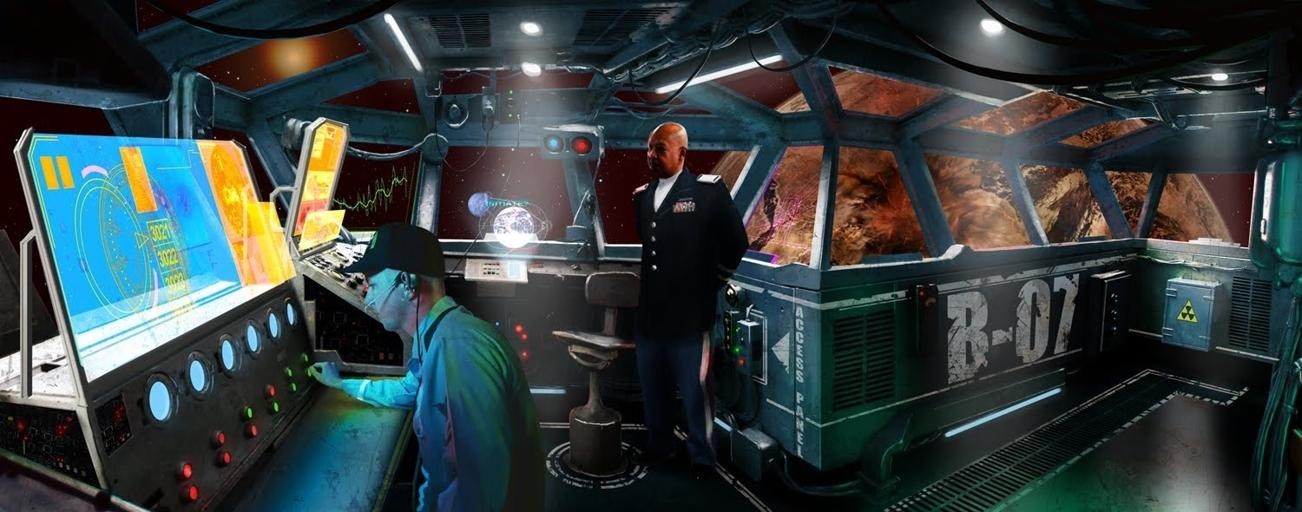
top-left (334, 222), bottom-right (446, 278)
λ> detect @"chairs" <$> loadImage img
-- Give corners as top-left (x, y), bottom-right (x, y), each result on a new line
top-left (552, 272), bottom-right (640, 475)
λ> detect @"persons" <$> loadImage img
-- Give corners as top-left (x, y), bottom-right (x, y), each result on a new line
top-left (628, 121), bottom-right (750, 482)
top-left (297, 220), bottom-right (550, 512)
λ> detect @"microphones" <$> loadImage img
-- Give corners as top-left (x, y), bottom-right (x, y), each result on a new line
top-left (364, 272), bottom-right (402, 314)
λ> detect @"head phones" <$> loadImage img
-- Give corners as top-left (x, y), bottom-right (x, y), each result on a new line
top-left (397, 272), bottom-right (425, 306)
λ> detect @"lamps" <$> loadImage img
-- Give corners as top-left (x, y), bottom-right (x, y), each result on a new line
top-left (542, 123), bottom-right (604, 163)
